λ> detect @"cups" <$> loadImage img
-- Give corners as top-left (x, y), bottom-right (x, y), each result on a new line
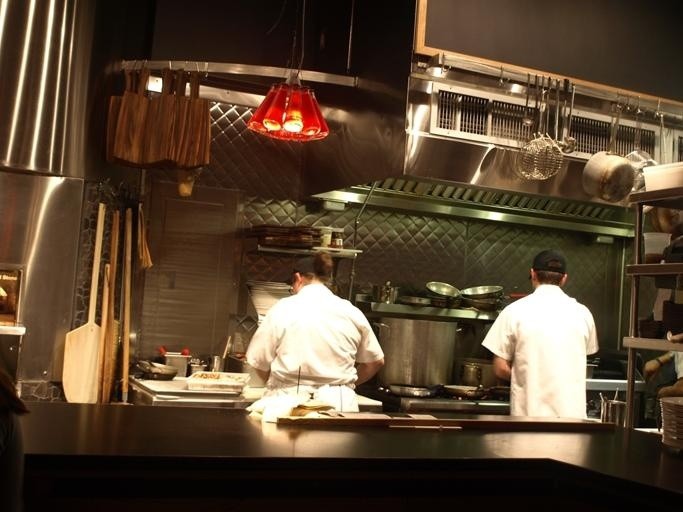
top-left (601, 400), bottom-right (626, 428)
top-left (208, 356), bottom-right (226, 372)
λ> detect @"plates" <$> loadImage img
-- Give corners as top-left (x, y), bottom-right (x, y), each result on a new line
top-left (661, 397), bottom-right (682, 447)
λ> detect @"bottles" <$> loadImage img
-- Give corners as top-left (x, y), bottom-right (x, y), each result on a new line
top-left (331, 228), bottom-right (344, 249)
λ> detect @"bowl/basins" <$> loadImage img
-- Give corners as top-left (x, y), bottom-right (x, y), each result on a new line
top-left (426, 281), bottom-right (505, 311)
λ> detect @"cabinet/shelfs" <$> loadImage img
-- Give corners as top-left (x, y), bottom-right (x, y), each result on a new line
top-left (622, 186), bottom-right (683, 429)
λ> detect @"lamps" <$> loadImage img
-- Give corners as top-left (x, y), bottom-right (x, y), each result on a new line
top-left (246, 0), bottom-right (329, 143)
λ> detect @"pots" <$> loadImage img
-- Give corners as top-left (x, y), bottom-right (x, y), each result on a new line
top-left (375, 315), bottom-right (497, 400)
top-left (135, 359), bottom-right (177, 381)
top-left (581, 104), bottom-right (635, 204)
top-left (373, 284), bottom-right (401, 304)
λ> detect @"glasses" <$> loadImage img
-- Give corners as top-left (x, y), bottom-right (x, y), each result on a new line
top-left (287, 279), bottom-right (299, 296)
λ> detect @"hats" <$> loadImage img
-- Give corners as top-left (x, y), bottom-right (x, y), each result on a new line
top-left (284, 254), bottom-right (328, 286)
top-left (527, 248), bottom-right (567, 279)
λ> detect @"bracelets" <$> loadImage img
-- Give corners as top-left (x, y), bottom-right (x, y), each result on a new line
top-left (656, 358), bottom-right (664, 367)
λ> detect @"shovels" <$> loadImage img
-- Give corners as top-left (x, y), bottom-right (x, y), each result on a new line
top-left (62, 203), bottom-right (105, 403)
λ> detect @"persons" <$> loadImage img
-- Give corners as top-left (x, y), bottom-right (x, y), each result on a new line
top-left (244, 251), bottom-right (385, 394)
top-left (644, 351), bottom-right (683, 397)
top-left (480, 249), bottom-right (599, 417)
